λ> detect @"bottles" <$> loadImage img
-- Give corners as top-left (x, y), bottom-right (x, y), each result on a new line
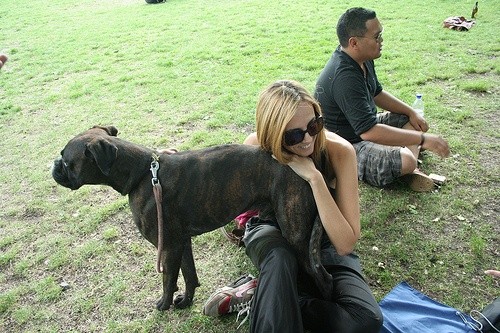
top-left (471, 2), bottom-right (479, 18)
top-left (413, 94), bottom-right (424, 117)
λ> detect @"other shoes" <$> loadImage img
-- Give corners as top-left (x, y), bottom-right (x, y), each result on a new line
top-left (400, 168), bottom-right (434, 192)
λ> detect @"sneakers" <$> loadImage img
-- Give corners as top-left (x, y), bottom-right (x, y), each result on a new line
top-left (202, 271), bottom-right (258, 332)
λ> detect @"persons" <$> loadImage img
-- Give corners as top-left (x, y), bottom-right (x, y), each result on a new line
top-left (475, 270), bottom-right (500, 333)
top-left (312, 6), bottom-right (450, 192)
top-left (202, 79), bottom-right (383, 333)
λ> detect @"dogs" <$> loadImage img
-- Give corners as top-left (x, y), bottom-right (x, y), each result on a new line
top-left (52, 125), bottom-right (333, 311)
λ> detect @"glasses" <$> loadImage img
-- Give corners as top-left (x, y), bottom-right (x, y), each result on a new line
top-left (350, 27), bottom-right (384, 43)
top-left (283, 108), bottom-right (324, 146)
